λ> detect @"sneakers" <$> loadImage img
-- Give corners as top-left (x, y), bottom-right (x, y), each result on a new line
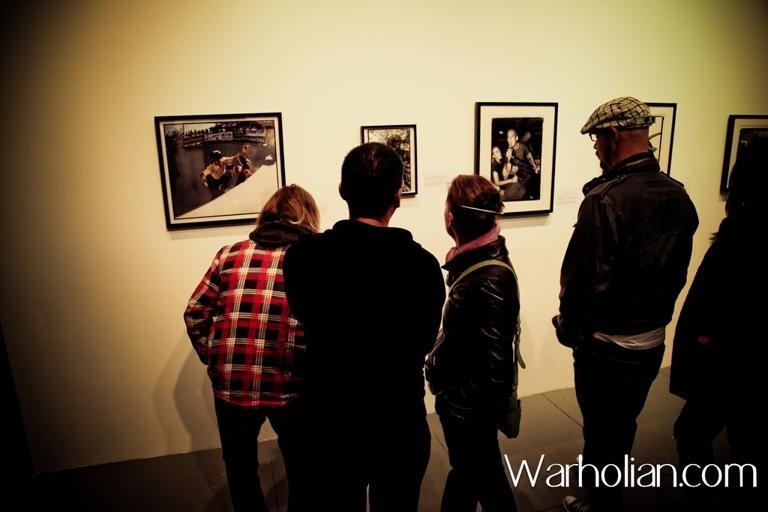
top-left (564, 453), bottom-right (594, 511)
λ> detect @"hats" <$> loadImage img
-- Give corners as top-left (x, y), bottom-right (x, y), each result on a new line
top-left (580, 98), bottom-right (656, 132)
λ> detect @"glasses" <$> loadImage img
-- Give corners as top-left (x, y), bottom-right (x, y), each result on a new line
top-left (589, 131), bottom-right (600, 142)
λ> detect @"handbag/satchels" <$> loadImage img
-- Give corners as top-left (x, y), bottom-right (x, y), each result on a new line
top-left (498, 367), bottom-right (521, 439)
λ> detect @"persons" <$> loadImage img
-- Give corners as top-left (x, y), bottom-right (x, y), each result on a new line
top-left (284, 141), bottom-right (446, 512)
top-left (184, 183), bottom-right (321, 511)
top-left (553, 97), bottom-right (699, 512)
top-left (200, 143), bottom-right (254, 198)
top-left (669, 141), bottom-right (767, 511)
top-left (491, 129), bottom-right (541, 200)
top-left (424, 175), bottom-right (526, 512)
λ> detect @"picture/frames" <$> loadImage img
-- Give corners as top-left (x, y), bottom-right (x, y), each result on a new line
top-left (361, 123), bottom-right (418, 199)
top-left (474, 101), bottom-right (558, 219)
top-left (153, 111), bottom-right (287, 231)
top-left (645, 102), bottom-right (677, 174)
top-left (720, 115), bottom-right (768, 191)
